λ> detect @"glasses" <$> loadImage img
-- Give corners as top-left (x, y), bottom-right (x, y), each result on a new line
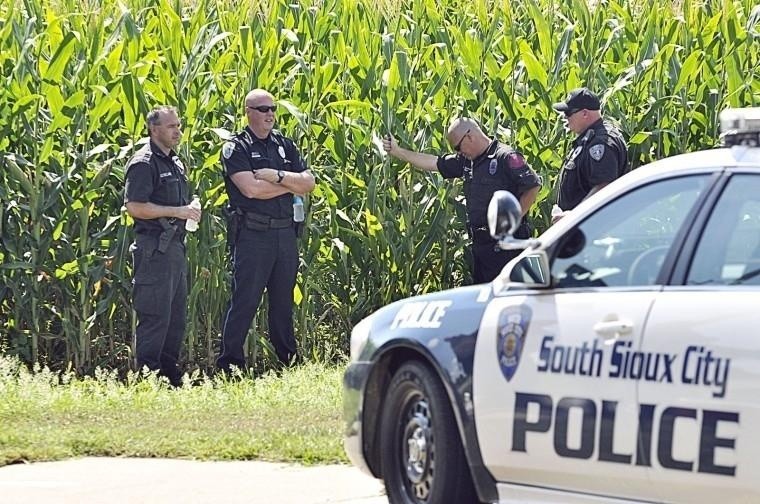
top-left (249, 105), bottom-right (278, 112)
top-left (564, 108), bottom-right (583, 118)
top-left (454, 128), bottom-right (471, 153)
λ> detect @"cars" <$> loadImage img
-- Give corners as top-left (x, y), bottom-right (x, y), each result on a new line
top-left (341, 107), bottom-right (760, 504)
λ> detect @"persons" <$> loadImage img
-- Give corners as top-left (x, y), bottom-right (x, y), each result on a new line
top-left (124, 105), bottom-right (201, 390)
top-left (215, 90), bottom-right (315, 383)
top-left (551, 89), bottom-right (628, 225)
top-left (382, 117), bottom-right (542, 283)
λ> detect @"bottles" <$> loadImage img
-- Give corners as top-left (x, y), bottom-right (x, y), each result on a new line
top-left (185, 196), bottom-right (202, 233)
top-left (550, 202), bottom-right (564, 217)
top-left (293, 190), bottom-right (305, 222)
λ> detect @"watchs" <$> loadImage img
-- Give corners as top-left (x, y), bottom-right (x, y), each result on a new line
top-left (277, 169), bottom-right (285, 184)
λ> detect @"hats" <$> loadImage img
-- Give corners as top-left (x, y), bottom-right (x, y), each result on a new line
top-left (553, 88), bottom-right (599, 112)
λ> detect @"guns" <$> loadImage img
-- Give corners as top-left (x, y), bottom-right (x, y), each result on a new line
top-left (157, 217), bottom-right (178, 254)
top-left (223, 204), bottom-right (238, 254)
